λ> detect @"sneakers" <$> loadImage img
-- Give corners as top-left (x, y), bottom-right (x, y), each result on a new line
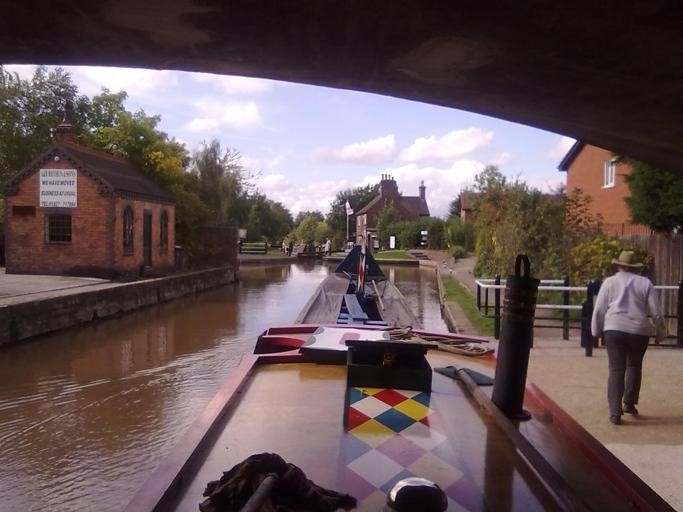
top-left (623, 403), bottom-right (638, 415)
top-left (609, 415), bottom-right (621, 424)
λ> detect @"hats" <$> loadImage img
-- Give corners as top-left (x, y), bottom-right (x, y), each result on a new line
top-left (611, 251), bottom-right (644, 267)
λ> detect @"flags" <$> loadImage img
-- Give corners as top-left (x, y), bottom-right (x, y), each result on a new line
top-left (346, 200), bottom-right (352, 216)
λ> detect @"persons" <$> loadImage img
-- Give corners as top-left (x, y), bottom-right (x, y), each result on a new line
top-left (325, 237), bottom-right (331, 255)
top-left (589, 250), bottom-right (668, 424)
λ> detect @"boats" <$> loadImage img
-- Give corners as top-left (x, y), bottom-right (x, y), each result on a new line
top-left (112, 235), bottom-right (676, 512)
